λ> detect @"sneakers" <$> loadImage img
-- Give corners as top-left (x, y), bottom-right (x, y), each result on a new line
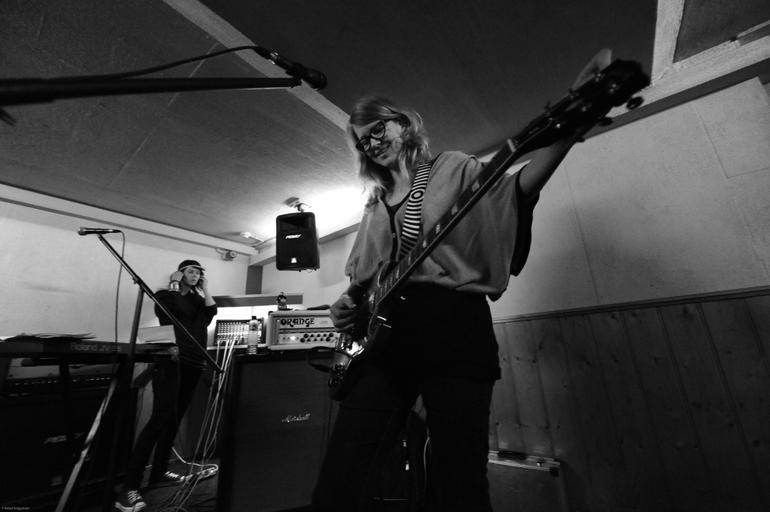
top-left (115, 490), bottom-right (147, 512)
top-left (148, 470), bottom-right (185, 489)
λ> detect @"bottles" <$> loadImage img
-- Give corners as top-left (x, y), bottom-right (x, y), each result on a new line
top-left (277, 292), bottom-right (287, 310)
top-left (246, 316), bottom-right (260, 354)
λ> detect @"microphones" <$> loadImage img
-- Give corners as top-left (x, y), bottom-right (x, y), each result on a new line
top-left (77, 227), bottom-right (121, 236)
top-left (254, 45), bottom-right (328, 91)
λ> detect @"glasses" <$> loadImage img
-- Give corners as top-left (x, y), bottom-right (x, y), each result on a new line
top-left (356, 119), bottom-right (391, 152)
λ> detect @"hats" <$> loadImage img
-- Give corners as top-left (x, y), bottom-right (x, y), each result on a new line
top-left (179, 260), bottom-right (201, 270)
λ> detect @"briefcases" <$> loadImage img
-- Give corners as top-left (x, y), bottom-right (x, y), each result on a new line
top-left (487, 450), bottom-right (566, 512)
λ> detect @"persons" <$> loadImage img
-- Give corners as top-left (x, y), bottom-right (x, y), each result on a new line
top-left (311, 44), bottom-right (615, 511)
top-left (110, 258), bottom-right (218, 512)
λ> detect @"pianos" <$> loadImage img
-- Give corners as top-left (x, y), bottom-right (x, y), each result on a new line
top-left (0, 340), bottom-right (179, 363)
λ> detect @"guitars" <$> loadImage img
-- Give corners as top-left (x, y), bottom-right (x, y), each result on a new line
top-left (328, 57), bottom-right (650, 401)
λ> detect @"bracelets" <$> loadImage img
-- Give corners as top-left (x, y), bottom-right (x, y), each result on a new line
top-left (168, 280), bottom-right (179, 283)
top-left (169, 284), bottom-right (181, 291)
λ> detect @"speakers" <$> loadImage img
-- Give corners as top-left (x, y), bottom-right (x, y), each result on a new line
top-left (216, 349), bottom-right (342, 512)
top-left (276, 212), bottom-right (320, 271)
top-left (0, 340), bottom-right (132, 512)
